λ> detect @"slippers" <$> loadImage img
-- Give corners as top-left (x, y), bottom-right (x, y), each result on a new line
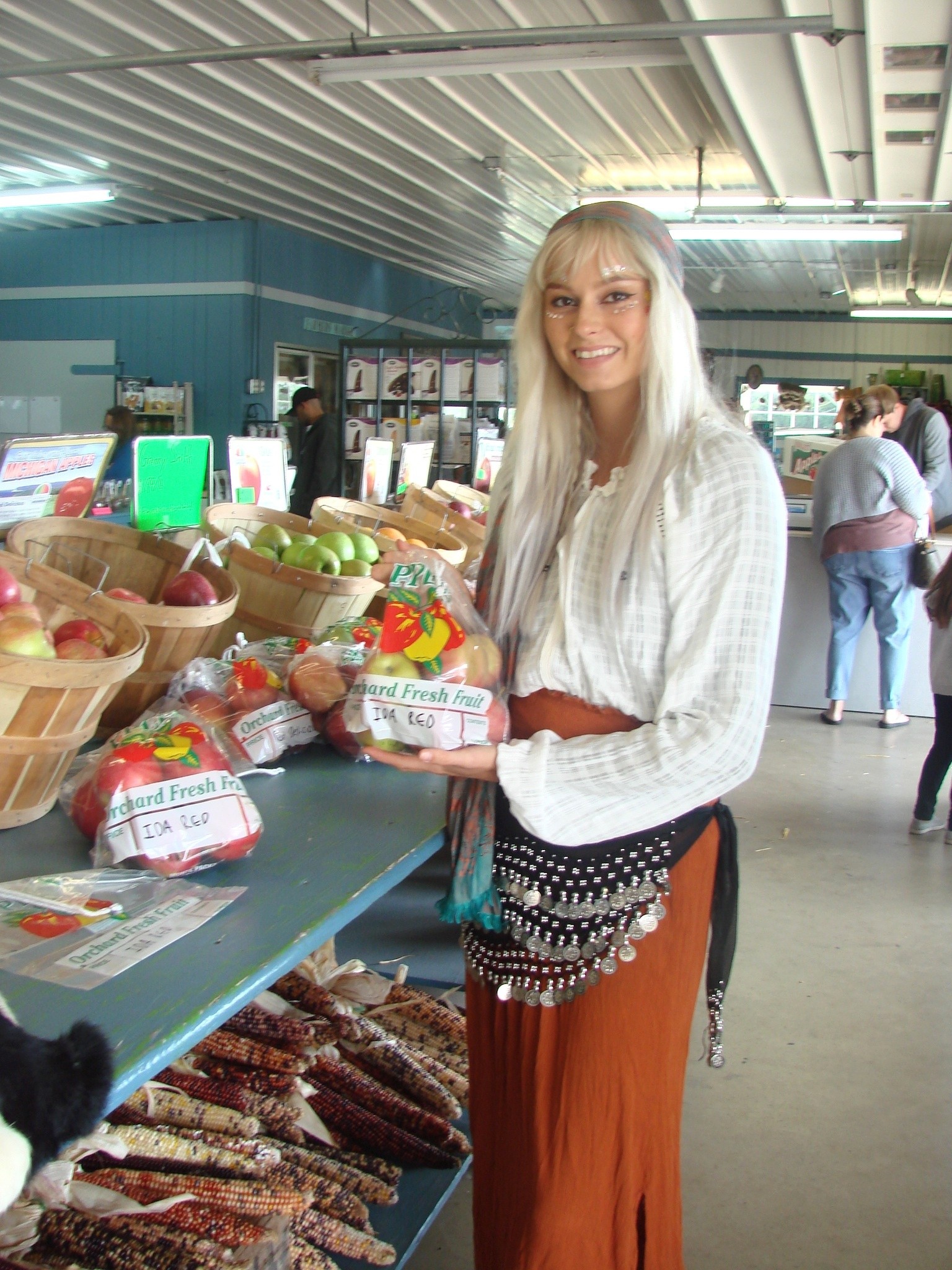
top-left (821, 711), bottom-right (842, 724)
top-left (878, 713), bottom-right (910, 728)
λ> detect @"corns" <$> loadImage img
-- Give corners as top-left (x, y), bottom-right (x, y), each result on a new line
top-left (24, 938), bottom-right (467, 1270)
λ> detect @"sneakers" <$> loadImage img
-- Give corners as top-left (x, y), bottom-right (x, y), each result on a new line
top-left (943, 830), bottom-right (952, 845)
top-left (910, 817), bottom-right (946, 834)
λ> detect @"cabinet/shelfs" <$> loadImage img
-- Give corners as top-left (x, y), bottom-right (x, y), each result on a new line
top-left (339, 285), bottom-right (519, 500)
top-left (0, 748), bottom-right (475, 1270)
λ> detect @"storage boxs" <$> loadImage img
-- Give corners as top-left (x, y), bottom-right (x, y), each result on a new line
top-left (115, 375), bottom-right (185, 414)
top-left (885, 361), bottom-right (925, 387)
top-left (782, 436), bottom-right (847, 483)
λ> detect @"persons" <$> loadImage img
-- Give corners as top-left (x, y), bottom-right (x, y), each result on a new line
top-left (288, 388), bottom-right (342, 518)
top-left (867, 384), bottom-right (951, 534)
top-left (353, 201), bottom-right (789, 1270)
top-left (809, 392), bottom-right (932, 729)
top-left (911, 548), bottom-right (952, 851)
top-left (101, 406), bottom-right (140, 488)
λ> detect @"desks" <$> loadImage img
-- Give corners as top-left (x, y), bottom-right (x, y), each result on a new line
top-left (769, 529), bottom-right (951, 718)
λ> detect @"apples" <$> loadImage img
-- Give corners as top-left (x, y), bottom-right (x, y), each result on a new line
top-left (1, 500), bottom-right (510, 875)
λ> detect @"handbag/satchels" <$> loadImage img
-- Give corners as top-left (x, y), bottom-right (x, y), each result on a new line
top-left (913, 510), bottom-right (940, 590)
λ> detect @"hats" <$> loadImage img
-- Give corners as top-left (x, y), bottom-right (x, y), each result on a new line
top-left (285, 387), bottom-right (321, 417)
